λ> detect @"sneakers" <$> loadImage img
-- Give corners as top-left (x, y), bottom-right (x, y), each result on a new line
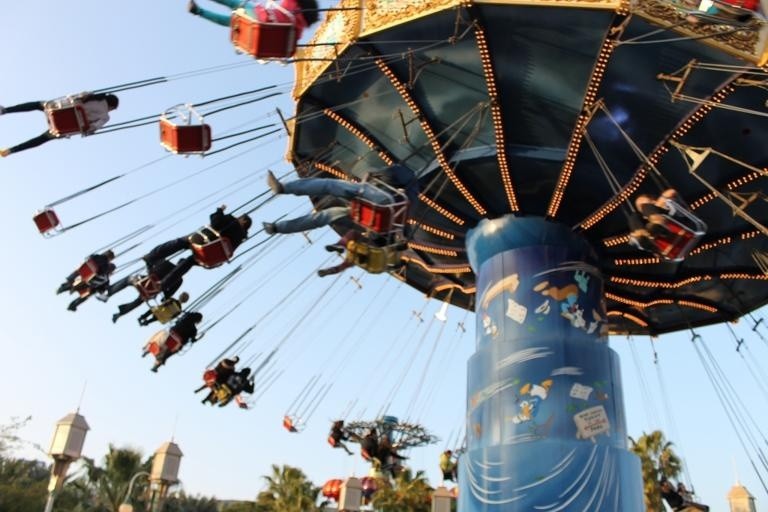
top-left (188, 0), bottom-right (196, 12)
top-left (326, 244), bottom-right (345, 254)
top-left (267, 170), bottom-right (283, 195)
top-left (264, 223), bottom-right (275, 234)
top-left (318, 269), bottom-right (334, 277)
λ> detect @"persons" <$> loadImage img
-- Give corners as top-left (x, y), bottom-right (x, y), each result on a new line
top-left (330, 421), bottom-right (410, 473)
top-left (316, 222), bottom-right (412, 278)
top-left (143, 204), bottom-right (252, 292)
top-left (439, 449), bottom-right (458, 483)
top-left (186, 0), bottom-right (319, 41)
top-left (676, 482), bottom-right (696, 502)
top-left (262, 162), bottom-right (420, 235)
top-left (53, 248), bottom-right (256, 410)
top-left (0, 90), bottom-right (119, 158)
top-left (627, 188), bottom-right (697, 255)
top-left (660, 480), bottom-right (709, 512)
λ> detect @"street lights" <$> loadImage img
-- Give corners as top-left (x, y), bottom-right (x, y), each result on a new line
top-left (431, 486), bottom-right (454, 511)
top-left (43, 377), bottom-right (91, 512)
top-left (728, 484), bottom-right (755, 512)
top-left (144, 437), bottom-right (183, 512)
top-left (339, 480), bottom-right (361, 509)
